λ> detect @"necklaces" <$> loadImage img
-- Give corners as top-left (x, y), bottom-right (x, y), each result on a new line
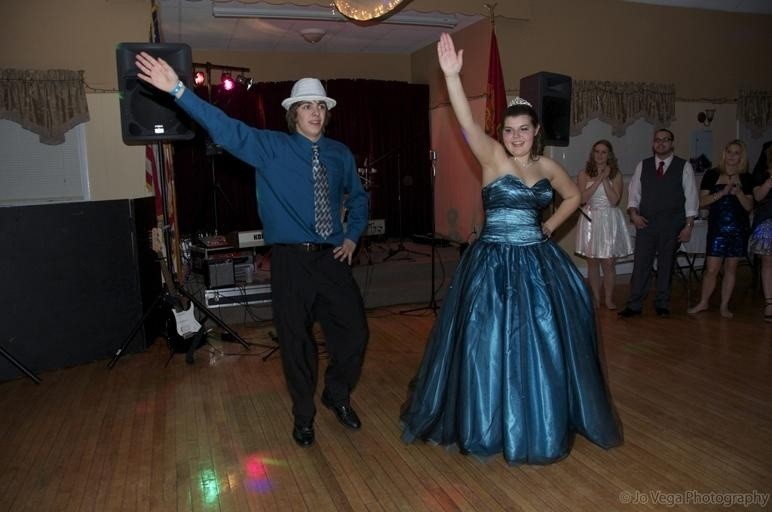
top-left (513, 156), bottom-right (533, 170)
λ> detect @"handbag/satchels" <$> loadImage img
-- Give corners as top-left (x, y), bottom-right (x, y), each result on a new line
top-left (689, 153), bottom-right (712, 173)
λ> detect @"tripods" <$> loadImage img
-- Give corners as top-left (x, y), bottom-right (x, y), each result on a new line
top-left (104, 143), bottom-right (248, 372)
top-left (400, 219), bottom-right (448, 319)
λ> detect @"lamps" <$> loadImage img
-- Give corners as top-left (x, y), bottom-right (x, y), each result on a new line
top-left (194, 66), bottom-right (256, 96)
top-left (298, 28), bottom-right (328, 45)
top-left (697, 108), bottom-right (716, 127)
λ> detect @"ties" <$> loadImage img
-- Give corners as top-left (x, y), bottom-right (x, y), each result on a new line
top-left (656, 162), bottom-right (664, 181)
top-left (312, 146), bottom-right (335, 239)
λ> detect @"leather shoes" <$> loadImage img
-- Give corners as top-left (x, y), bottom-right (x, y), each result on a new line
top-left (761, 298), bottom-right (772, 321)
top-left (293, 423), bottom-right (314, 447)
top-left (321, 393), bottom-right (361, 430)
top-left (617, 307), bottom-right (642, 317)
top-left (656, 307), bottom-right (669, 316)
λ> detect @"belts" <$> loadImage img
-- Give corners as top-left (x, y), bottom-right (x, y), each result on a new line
top-left (272, 242), bottom-right (334, 252)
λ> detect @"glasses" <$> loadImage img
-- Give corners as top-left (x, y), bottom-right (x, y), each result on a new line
top-left (655, 137), bottom-right (671, 141)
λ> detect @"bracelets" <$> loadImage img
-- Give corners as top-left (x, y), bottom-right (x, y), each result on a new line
top-left (686, 222), bottom-right (694, 230)
top-left (722, 188), bottom-right (728, 195)
top-left (713, 193), bottom-right (719, 200)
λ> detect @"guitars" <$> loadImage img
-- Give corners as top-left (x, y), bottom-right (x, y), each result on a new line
top-left (148, 227), bottom-right (207, 355)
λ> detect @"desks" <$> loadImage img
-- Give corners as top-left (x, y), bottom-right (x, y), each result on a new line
top-left (627, 216), bottom-right (758, 302)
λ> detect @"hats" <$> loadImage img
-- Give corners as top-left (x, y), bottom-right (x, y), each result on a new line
top-left (282, 78), bottom-right (337, 110)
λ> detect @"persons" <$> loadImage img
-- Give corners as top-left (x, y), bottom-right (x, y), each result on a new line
top-left (574, 139), bottom-right (634, 310)
top-left (687, 139), bottom-right (753, 319)
top-left (617, 128), bottom-right (698, 317)
top-left (746, 141), bottom-right (772, 324)
top-left (398, 32), bottom-right (626, 465)
top-left (136, 51), bottom-right (370, 448)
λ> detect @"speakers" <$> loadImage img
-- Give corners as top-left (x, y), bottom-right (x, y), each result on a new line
top-left (115, 42), bottom-right (193, 146)
top-left (518, 70), bottom-right (573, 146)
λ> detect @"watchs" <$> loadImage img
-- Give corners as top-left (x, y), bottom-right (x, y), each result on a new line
top-left (172, 81), bottom-right (184, 97)
